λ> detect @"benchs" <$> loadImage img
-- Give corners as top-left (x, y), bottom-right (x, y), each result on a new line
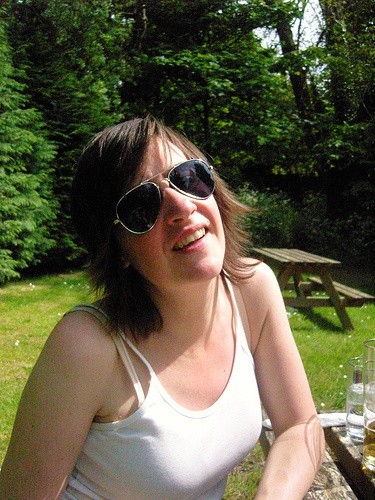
top-left (304, 276), bottom-right (375, 308)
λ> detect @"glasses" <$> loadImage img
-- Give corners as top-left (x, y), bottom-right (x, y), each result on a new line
top-left (113, 158), bottom-right (216, 235)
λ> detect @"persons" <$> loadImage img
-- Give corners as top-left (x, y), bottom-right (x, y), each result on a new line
top-left (0, 117), bottom-right (327, 500)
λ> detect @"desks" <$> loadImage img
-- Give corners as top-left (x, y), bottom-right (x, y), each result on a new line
top-left (251, 247), bottom-right (352, 334)
top-left (259, 408), bottom-right (375, 500)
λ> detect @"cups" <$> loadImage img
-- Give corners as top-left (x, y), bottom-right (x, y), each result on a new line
top-left (365, 360), bottom-right (375, 471)
top-left (363, 340), bottom-right (375, 360)
top-left (347, 357), bottom-right (364, 442)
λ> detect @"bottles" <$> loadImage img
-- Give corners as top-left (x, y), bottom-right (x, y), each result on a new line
top-left (347, 371), bottom-right (365, 394)
